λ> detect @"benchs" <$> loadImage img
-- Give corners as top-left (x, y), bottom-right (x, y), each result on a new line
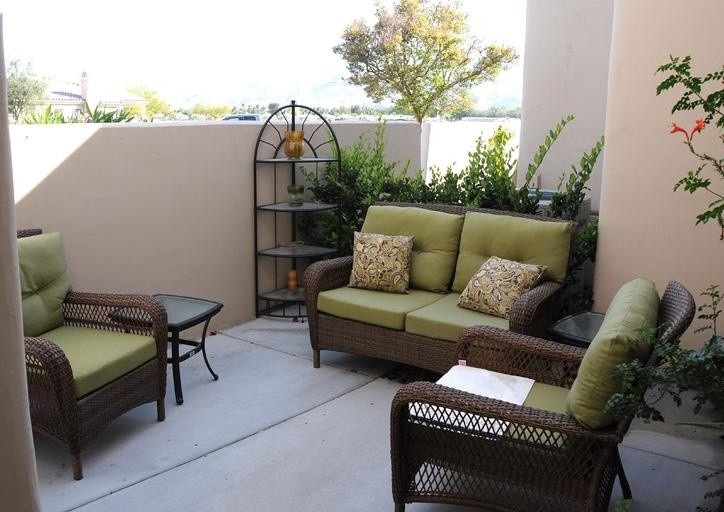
top-left (304, 200), bottom-right (576, 370)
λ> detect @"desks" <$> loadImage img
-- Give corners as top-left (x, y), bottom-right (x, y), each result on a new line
top-left (108, 293), bottom-right (224, 405)
top-left (550, 305), bottom-right (606, 345)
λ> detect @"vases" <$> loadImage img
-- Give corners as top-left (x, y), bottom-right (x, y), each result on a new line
top-left (286, 269), bottom-right (298, 294)
top-left (286, 184), bottom-right (304, 207)
top-left (282, 131), bottom-right (304, 160)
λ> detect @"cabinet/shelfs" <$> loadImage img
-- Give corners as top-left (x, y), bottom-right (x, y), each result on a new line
top-left (251, 100), bottom-right (342, 322)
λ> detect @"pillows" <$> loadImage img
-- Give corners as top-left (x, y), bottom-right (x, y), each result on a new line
top-left (347, 230), bottom-right (416, 295)
top-left (456, 255), bottom-right (549, 320)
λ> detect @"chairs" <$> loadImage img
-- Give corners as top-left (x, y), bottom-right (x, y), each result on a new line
top-left (15, 227), bottom-right (168, 481)
top-left (388, 279), bottom-right (700, 512)
